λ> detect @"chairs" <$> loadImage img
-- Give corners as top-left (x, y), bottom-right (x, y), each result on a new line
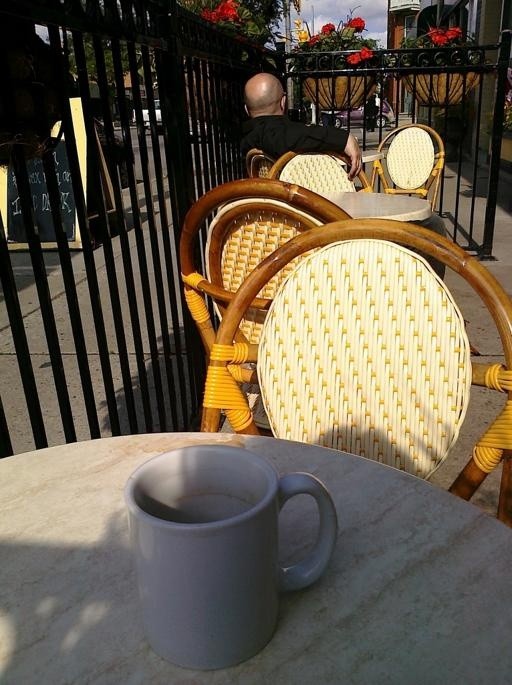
top-left (243, 146), bottom-right (276, 180)
top-left (180, 177), bottom-right (354, 430)
top-left (266, 149), bottom-right (374, 204)
top-left (195, 217), bottom-right (512, 532)
top-left (374, 123), bottom-right (446, 215)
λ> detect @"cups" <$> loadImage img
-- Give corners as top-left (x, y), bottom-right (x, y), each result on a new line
top-left (125, 446), bottom-right (338, 669)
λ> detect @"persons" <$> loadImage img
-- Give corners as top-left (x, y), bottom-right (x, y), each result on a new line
top-left (241, 72), bottom-right (363, 182)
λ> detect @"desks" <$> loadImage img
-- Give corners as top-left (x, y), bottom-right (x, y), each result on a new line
top-left (359, 150), bottom-right (386, 164)
top-left (314, 192), bottom-right (432, 226)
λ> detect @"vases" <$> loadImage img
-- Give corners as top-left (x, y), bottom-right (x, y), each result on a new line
top-left (403, 70), bottom-right (481, 107)
top-left (304, 72), bottom-right (378, 111)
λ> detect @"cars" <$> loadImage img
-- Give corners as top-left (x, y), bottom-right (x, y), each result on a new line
top-left (132, 100), bottom-right (162, 134)
top-left (334, 98), bottom-right (395, 129)
top-left (95, 118), bottom-right (135, 189)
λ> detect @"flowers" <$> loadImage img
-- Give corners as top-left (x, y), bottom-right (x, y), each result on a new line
top-left (392, 25), bottom-right (488, 65)
top-left (290, 6), bottom-right (383, 66)
top-left (200, 0), bottom-right (270, 44)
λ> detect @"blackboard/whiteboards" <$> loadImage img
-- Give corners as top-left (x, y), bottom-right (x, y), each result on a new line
top-left (0, 97), bottom-right (88, 251)
